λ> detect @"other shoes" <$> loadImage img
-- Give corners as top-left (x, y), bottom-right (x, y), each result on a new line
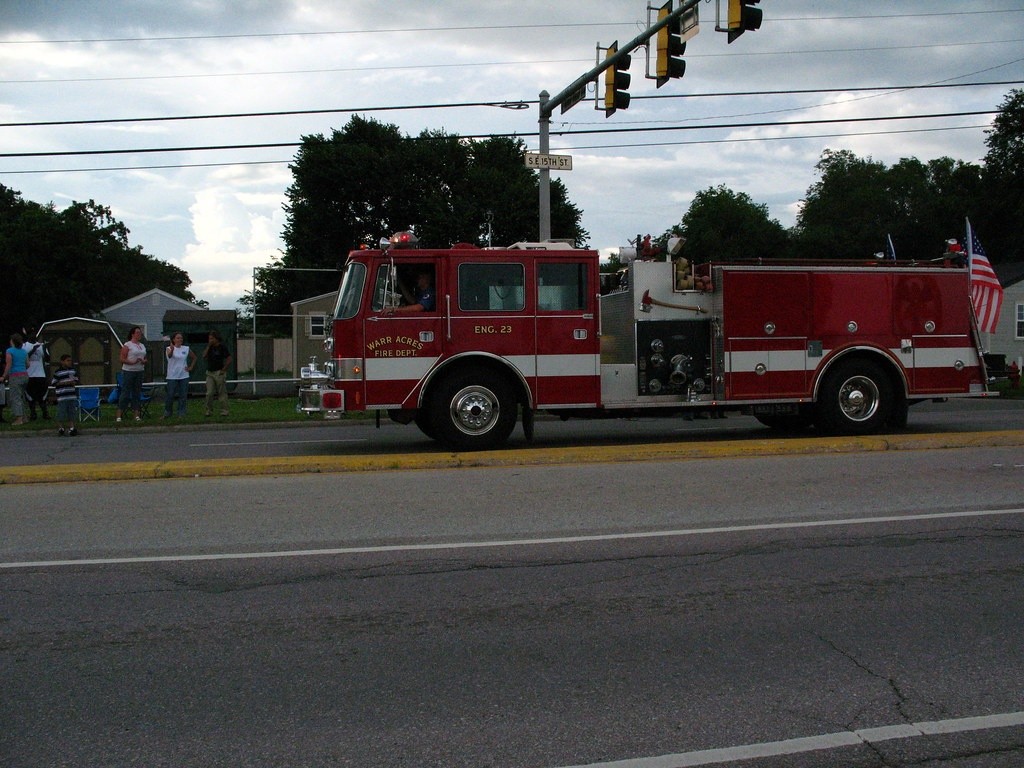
top-left (57, 428), bottom-right (64, 436)
top-left (206, 412), bottom-right (211, 416)
top-left (0, 418), bottom-right (8, 423)
top-left (43, 415), bottom-right (51, 420)
top-left (11, 419), bottom-right (28, 425)
top-left (221, 412), bottom-right (229, 416)
top-left (116, 417), bottom-right (121, 422)
top-left (135, 416), bottom-right (141, 421)
top-left (68, 427), bottom-right (77, 436)
top-left (30, 415), bottom-right (37, 421)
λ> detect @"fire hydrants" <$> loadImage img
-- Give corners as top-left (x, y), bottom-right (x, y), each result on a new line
top-left (1008, 361), bottom-right (1020, 390)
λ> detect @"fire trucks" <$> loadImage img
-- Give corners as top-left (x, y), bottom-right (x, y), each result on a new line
top-left (292, 231), bottom-right (1004, 454)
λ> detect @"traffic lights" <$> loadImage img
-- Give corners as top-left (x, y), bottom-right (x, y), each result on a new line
top-left (655, 0), bottom-right (688, 79)
top-left (604, 40), bottom-right (631, 117)
top-left (727, 0), bottom-right (763, 30)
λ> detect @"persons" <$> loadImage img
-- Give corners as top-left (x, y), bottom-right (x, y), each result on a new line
top-left (202, 331), bottom-right (233, 416)
top-left (380, 270), bottom-right (436, 317)
top-left (0, 327), bottom-right (81, 436)
top-left (116, 326), bottom-right (147, 422)
top-left (162, 332), bottom-right (197, 420)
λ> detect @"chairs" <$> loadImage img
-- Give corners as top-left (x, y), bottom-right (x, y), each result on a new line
top-left (76, 387), bottom-right (104, 422)
top-left (108, 372), bottom-right (157, 419)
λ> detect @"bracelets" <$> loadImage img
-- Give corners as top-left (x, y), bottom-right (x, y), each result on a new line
top-left (391, 306), bottom-right (394, 312)
top-left (2, 376), bottom-right (5, 379)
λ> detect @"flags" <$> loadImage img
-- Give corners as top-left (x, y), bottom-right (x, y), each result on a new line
top-left (885, 235), bottom-right (896, 260)
top-left (960, 219), bottom-right (1004, 334)
